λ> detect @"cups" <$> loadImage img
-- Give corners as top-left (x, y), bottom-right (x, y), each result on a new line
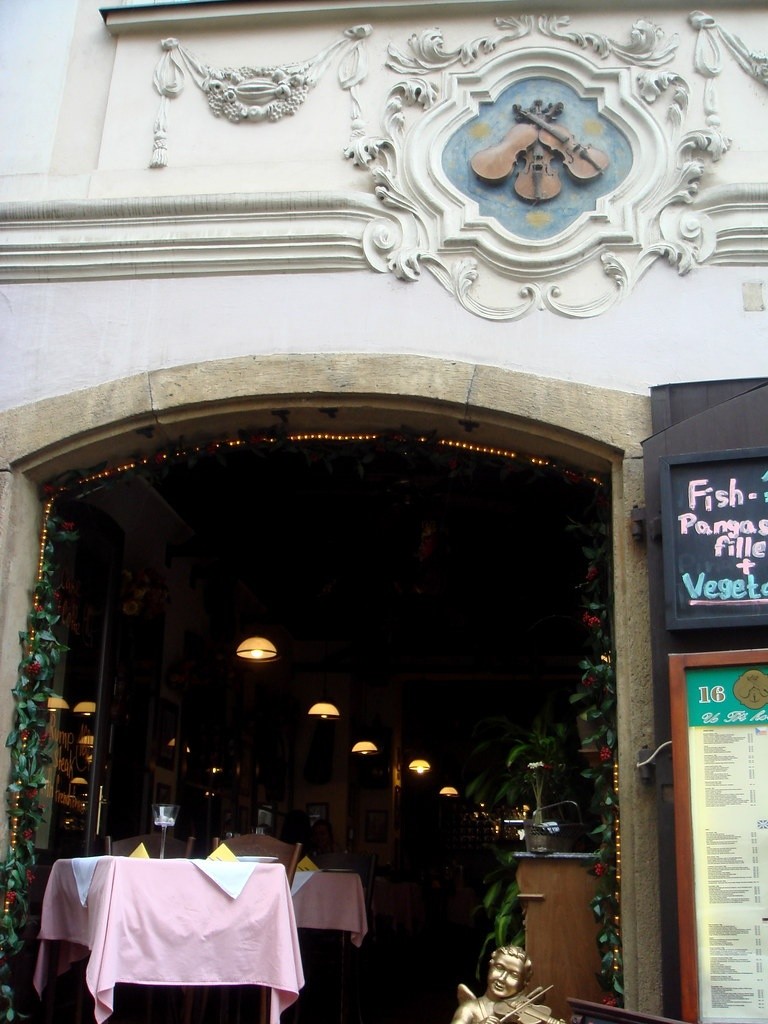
top-left (256, 828), bottom-right (263, 834)
top-left (226, 832), bottom-right (232, 838)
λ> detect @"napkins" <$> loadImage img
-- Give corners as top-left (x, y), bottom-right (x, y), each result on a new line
top-left (190, 858), bottom-right (260, 899)
top-left (71, 856), bottom-right (101, 907)
top-left (291, 872), bottom-right (314, 897)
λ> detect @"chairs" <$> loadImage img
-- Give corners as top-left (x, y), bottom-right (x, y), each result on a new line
top-left (73, 834), bottom-right (195, 1023)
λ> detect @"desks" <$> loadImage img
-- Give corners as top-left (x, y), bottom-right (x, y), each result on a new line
top-left (291, 871), bottom-right (368, 1024)
top-left (33, 856), bottom-right (305, 1024)
top-left (369, 883), bottom-right (425, 933)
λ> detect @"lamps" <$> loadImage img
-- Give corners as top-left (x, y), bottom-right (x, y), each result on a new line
top-left (236, 636), bottom-right (278, 662)
top-left (351, 687), bottom-right (379, 754)
top-left (306, 641), bottom-right (340, 719)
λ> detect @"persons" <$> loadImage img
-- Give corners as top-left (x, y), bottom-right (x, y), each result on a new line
top-left (280, 810), bottom-right (315, 859)
top-left (450, 944), bottom-right (566, 1024)
top-left (310, 818), bottom-right (342, 855)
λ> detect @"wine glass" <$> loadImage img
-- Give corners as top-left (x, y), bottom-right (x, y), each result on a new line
top-left (152, 803), bottom-right (180, 859)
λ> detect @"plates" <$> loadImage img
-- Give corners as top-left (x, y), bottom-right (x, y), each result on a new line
top-left (327, 869), bottom-right (354, 872)
top-left (236, 856), bottom-right (278, 863)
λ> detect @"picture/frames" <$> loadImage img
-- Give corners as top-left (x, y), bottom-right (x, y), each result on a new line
top-left (305, 802), bottom-right (328, 825)
top-left (151, 697), bottom-right (180, 772)
top-left (364, 809), bottom-right (389, 843)
top-left (156, 782), bottom-right (172, 804)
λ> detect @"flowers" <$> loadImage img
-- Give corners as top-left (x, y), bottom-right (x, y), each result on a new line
top-left (520, 761), bottom-right (553, 817)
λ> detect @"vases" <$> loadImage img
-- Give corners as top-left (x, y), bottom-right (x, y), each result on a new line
top-left (522, 820), bottom-right (559, 853)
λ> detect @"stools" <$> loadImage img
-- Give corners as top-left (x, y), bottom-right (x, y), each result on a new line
top-left (182, 834), bottom-right (303, 1024)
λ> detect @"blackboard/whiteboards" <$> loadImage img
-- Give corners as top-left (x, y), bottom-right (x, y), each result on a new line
top-left (656, 446), bottom-right (768, 632)
